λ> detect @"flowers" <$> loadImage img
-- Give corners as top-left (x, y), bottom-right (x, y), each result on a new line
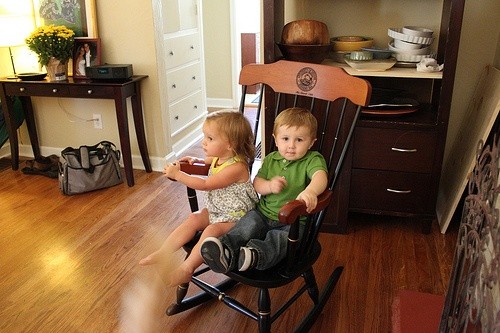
top-left (25, 25), bottom-right (76, 71)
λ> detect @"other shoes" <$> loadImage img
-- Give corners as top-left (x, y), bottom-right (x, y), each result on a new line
top-left (238, 247), bottom-right (256, 272)
top-left (201, 238), bottom-right (228, 274)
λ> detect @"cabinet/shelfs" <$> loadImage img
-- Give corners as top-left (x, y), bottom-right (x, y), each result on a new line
top-left (265, 77), bottom-right (443, 236)
top-left (164, 28), bottom-right (208, 137)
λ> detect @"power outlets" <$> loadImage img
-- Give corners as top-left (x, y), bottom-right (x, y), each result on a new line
top-left (93, 114), bottom-right (102, 129)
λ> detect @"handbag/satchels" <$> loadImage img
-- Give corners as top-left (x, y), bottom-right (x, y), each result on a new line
top-left (58, 140), bottom-right (123, 195)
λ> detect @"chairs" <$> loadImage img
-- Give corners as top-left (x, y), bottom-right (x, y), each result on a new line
top-left (165, 60), bottom-right (372, 333)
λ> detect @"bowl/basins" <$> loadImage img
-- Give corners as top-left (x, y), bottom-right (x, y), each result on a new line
top-left (332, 25), bottom-right (434, 71)
top-left (281, 19), bottom-right (329, 45)
top-left (274, 40), bottom-right (335, 64)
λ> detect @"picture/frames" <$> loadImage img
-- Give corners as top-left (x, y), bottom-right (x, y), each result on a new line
top-left (30, 0), bottom-right (98, 76)
top-left (73, 37), bottom-right (101, 80)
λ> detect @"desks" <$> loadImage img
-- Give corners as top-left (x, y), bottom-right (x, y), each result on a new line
top-left (0, 75), bottom-right (152, 187)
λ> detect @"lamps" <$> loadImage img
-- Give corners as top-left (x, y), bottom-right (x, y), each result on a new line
top-left (0, 16), bottom-right (32, 79)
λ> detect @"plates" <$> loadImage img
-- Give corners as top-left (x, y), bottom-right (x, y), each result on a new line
top-left (396, 61), bottom-right (418, 68)
top-left (16, 72), bottom-right (48, 80)
top-left (359, 107), bottom-right (419, 117)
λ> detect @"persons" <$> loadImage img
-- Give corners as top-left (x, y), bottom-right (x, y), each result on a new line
top-left (138, 106), bottom-right (260, 286)
top-left (199, 107), bottom-right (327, 273)
top-left (74, 43), bottom-right (96, 76)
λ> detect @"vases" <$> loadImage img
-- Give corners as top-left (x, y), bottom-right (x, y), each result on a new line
top-left (44, 57), bottom-right (66, 81)
